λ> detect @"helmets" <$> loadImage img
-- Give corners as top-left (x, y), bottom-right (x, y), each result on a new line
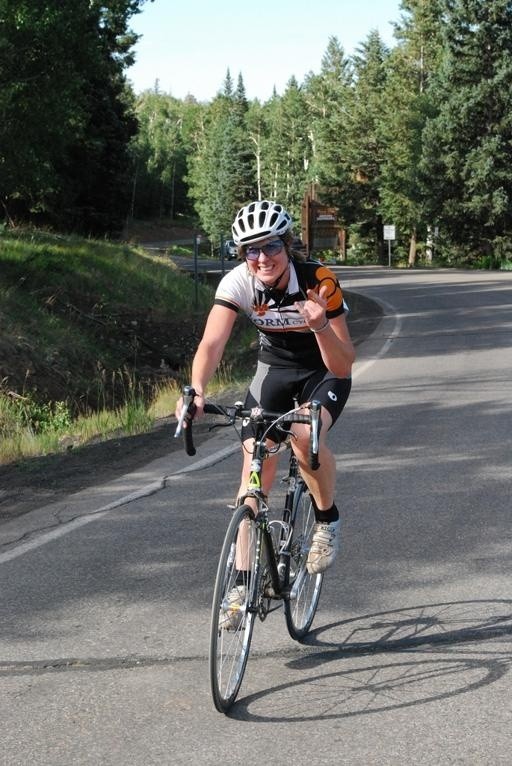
top-left (230, 197), bottom-right (292, 250)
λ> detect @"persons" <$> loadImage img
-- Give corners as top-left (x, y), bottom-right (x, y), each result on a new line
top-left (174, 198), bottom-right (356, 633)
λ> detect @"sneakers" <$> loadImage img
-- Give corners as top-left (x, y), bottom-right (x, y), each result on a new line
top-left (305, 517), bottom-right (343, 576)
top-left (213, 584), bottom-right (255, 632)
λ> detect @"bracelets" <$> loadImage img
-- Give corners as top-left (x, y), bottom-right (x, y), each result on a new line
top-left (308, 320), bottom-right (330, 334)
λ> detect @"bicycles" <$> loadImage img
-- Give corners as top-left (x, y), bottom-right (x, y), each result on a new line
top-left (171, 389), bottom-right (331, 719)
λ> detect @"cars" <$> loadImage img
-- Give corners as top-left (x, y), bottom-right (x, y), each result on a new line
top-left (224, 240), bottom-right (240, 262)
top-left (290, 237), bottom-right (310, 261)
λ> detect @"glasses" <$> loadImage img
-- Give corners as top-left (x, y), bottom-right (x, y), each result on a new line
top-left (240, 239), bottom-right (290, 262)
